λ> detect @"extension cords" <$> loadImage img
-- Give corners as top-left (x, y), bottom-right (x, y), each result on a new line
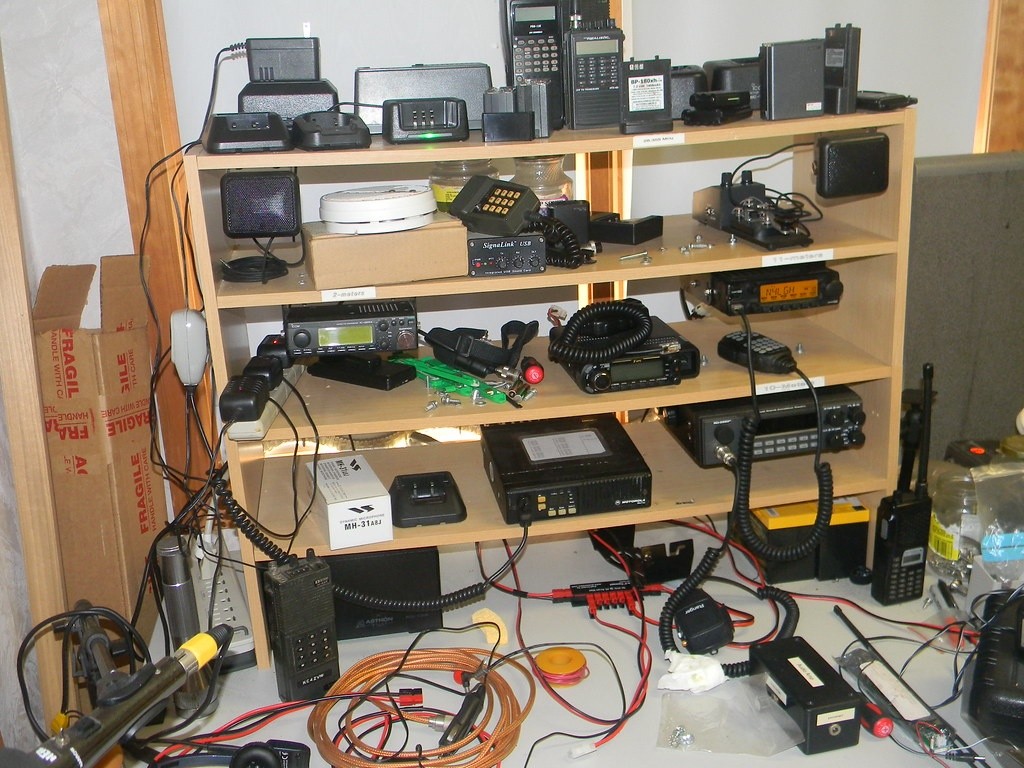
top-left (184, 529), bottom-right (258, 675)
top-left (227, 354), bottom-right (307, 441)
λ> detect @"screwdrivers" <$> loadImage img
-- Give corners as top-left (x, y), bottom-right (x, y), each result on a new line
top-left (854, 693), bottom-right (894, 739)
top-left (520, 354), bottom-right (545, 385)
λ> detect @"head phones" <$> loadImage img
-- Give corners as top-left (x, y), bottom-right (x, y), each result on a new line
top-left (148, 741), bottom-right (284, 768)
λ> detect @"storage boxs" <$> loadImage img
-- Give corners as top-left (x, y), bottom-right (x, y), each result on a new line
top-left (747, 635), bottom-right (865, 756)
top-left (724, 495), bottom-right (817, 585)
top-left (304, 454), bottom-right (392, 551)
top-left (256, 544), bottom-right (444, 649)
top-left (33, 255), bottom-right (168, 677)
top-left (811, 495), bottom-right (869, 579)
top-left (302, 211), bottom-right (471, 290)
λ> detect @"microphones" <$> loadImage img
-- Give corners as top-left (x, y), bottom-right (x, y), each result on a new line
top-left (0, 624), bottom-right (229, 768)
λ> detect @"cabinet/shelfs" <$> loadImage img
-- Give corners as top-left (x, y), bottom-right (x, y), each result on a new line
top-left (182, 105), bottom-right (917, 677)
